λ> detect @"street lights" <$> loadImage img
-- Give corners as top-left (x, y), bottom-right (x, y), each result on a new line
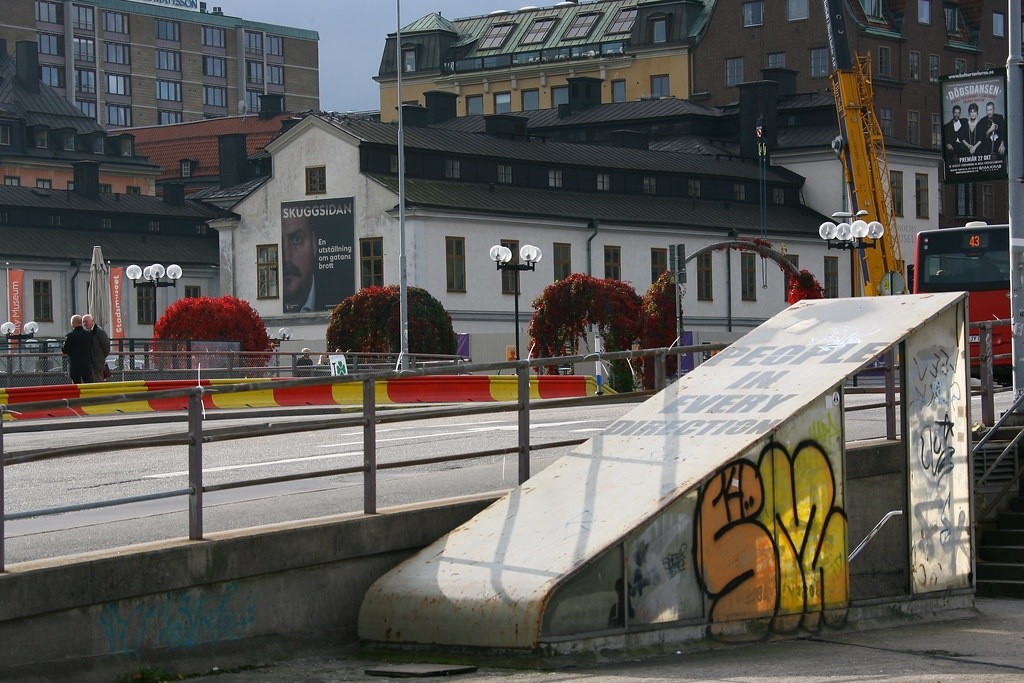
top-left (818, 219), bottom-right (885, 297)
top-left (126, 264), bottom-right (183, 337)
top-left (0, 321), bottom-right (40, 371)
top-left (489, 245), bottom-right (543, 376)
top-left (831, 209), bottom-right (869, 297)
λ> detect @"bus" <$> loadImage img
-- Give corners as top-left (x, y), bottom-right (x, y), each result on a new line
top-left (904, 221), bottom-right (1014, 386)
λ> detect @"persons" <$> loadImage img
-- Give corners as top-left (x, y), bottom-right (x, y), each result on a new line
top-left (281, 205), bottom-right (341, 313)
top-left (316, 348), bottom-right (353, 376)
top-left (62, 316), bottom-right (93, 384)
top-left (83, 315), bottom-right (111, 383)
top-left (978, 256), bottom-right (1001, 273)
top-left (297, 348), bottom-right (314, 377)
top-left (944, 102), bottom-right (1006, 161)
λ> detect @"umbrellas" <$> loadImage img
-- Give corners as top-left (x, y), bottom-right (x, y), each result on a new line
top-left (88, 246), bottom-right (108, 327)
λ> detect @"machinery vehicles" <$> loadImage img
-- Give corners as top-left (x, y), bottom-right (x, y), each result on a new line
top-left (820, 0), bottom-right (908, 300)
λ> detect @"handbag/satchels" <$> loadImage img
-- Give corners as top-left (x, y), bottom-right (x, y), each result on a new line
top-left (103, 364), bottom-right (112, 378)
